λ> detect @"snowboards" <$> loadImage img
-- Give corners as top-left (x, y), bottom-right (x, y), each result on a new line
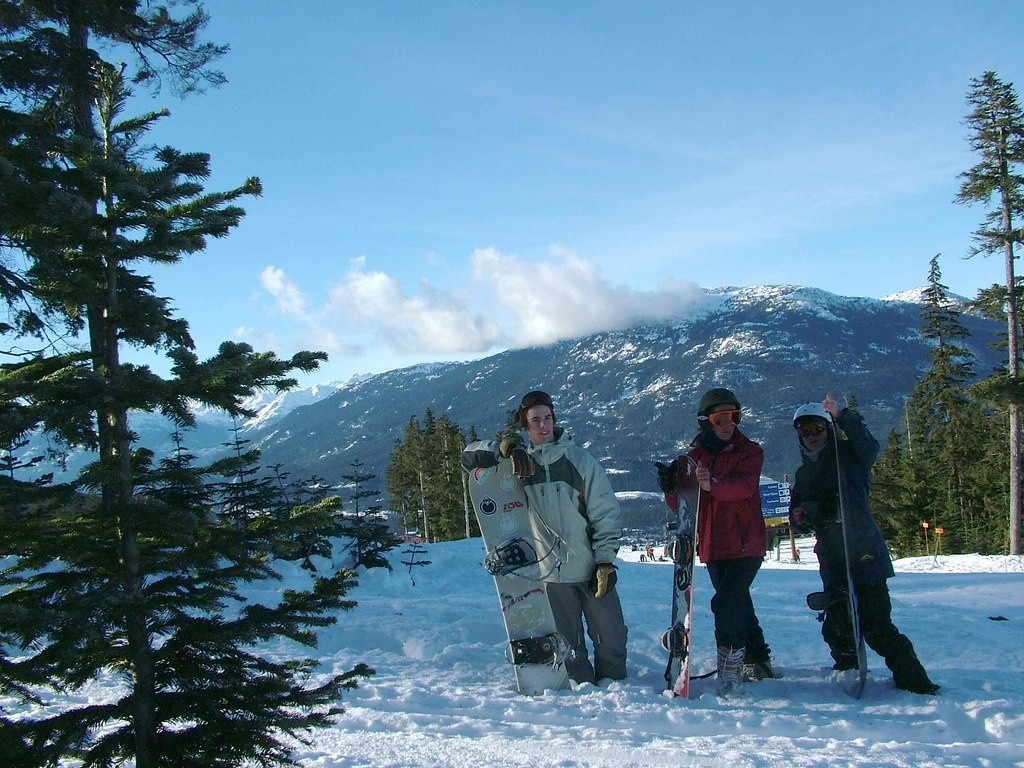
top-left (815, 412), bottom-right (868, 700)
top-left (660, 454), bottom-right (702, 699)
top-left (468, 456), bottom-right (572, 695)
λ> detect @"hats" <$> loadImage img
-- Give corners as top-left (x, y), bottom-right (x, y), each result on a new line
top-left (518, 391), bottom-right (552, 410)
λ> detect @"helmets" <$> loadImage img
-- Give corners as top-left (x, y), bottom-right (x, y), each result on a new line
top-left (792, 403), bottom-right (833, 429)
top-left (698, 388), bottom-right (740, 420)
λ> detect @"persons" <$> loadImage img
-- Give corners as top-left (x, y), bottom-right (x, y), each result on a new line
top-left (788, 394), bottom-right (941, 697)
top-left (654, 389), bottom-right (775, 683)
top-left (631, 537), bottom-right (669, 563)
top-left (795, 549), bottom-right (801, 562)
top-left (461, 392), bottom-right (628, 687)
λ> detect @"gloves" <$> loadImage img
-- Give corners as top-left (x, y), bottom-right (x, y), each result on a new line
top-left (499, 432), bottom-right (534, 481)
top-left (654, 460), bottom-right (680, 494)
top-left (594, 563), bottom-right (619, 599)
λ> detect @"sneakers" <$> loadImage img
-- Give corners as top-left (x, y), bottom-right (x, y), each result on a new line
top-left (717, 645), bottom-right (746, 699)
top-left (899, 668), bottom-right (940, 695)
top-left (741, 657), bottom-right (778, 682)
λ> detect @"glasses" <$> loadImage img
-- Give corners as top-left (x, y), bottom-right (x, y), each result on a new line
top-left (697, 410), bottom-right (742, 426)
top-left (796, 418), bottom-right (830, 440)
